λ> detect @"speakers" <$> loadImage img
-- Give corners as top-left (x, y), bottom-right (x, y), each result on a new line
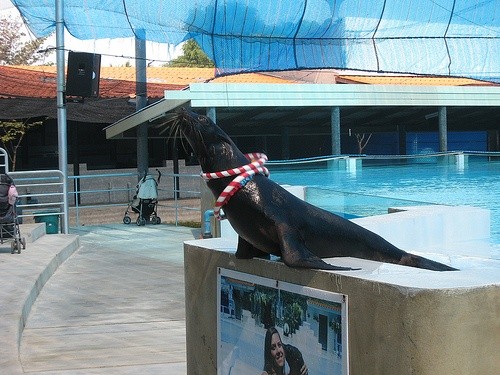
top-left (66, 52), bottom-right (102, 98)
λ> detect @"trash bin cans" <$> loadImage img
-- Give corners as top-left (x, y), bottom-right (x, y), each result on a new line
top-left (31, 209), bottom-right (60, 234)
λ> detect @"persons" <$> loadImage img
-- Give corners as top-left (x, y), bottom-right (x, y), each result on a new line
top-left (259, 327), bottom-right (309, 375)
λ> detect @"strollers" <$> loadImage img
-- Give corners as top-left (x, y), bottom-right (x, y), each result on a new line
top-left (123, 168), bottom-right (163, 226)
top-left (1, 173), bottom-right (27, 255)
